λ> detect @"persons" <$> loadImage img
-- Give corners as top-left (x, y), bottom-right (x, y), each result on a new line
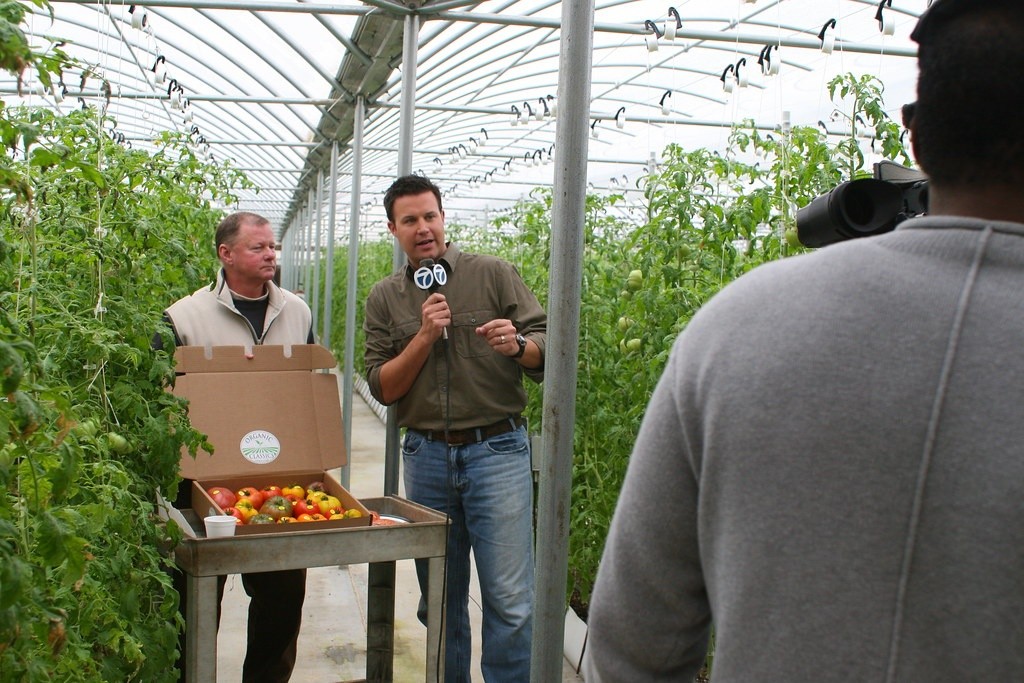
top-left (363, 174), bottom-right (547, 683)
top-left (583, 0), bottom-right (1024, 683)
top-left (148, 212), bottom-right (315, 683)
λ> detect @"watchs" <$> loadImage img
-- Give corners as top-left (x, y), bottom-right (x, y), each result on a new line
top-left (510, 333), bottom-right (527, 358)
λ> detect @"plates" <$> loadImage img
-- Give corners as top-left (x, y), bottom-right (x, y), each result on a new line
top-left (379, 514), bottom-right (413, 524)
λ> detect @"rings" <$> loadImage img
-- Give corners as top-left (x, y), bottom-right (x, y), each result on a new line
top-left (501, 335), bottom-right (506, 344)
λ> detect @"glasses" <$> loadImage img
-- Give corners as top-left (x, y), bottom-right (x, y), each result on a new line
top-left (899, 129), bottom-right (913, 160)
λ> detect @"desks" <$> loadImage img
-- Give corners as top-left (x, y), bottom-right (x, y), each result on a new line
top-left (159, 495), bottom-right (454, 683)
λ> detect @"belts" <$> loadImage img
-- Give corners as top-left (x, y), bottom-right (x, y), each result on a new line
top-left (417, 417), bottom-right (521, 443)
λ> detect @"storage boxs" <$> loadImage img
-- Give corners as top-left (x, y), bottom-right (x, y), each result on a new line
top-left (163, 344), bottom-right (373, 534)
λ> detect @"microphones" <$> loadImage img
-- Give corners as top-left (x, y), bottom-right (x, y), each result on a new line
top-left (414, 258), bottom-right (448, 344)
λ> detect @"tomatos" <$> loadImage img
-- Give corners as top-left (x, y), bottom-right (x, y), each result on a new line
top-left (207, 481), bottom-right (360, 526)
top-left (76, 415), bottom-right (139, 454)
top-left (618, 268), bottom-right (643, 349)
top-left (785, 227), bottom-right (802, 249)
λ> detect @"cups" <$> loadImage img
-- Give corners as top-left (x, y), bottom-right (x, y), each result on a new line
top-left (204, 516), bottom-right (237, 538)
